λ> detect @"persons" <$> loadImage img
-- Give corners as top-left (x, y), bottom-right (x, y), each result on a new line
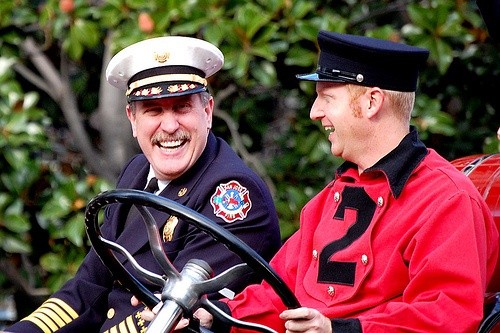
top-left (1, 35), bottom-right (281, 333)
top-left (131, 30), bottom-right (500, 333)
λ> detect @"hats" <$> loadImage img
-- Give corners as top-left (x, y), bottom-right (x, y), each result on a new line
top-left (105, 35), bottom-right (225, 100)
top-left (294, 29), bottom-right (430, 93)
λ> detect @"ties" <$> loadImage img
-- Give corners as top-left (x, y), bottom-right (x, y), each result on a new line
top-left (123, 176), bottom-right (159, 231)
